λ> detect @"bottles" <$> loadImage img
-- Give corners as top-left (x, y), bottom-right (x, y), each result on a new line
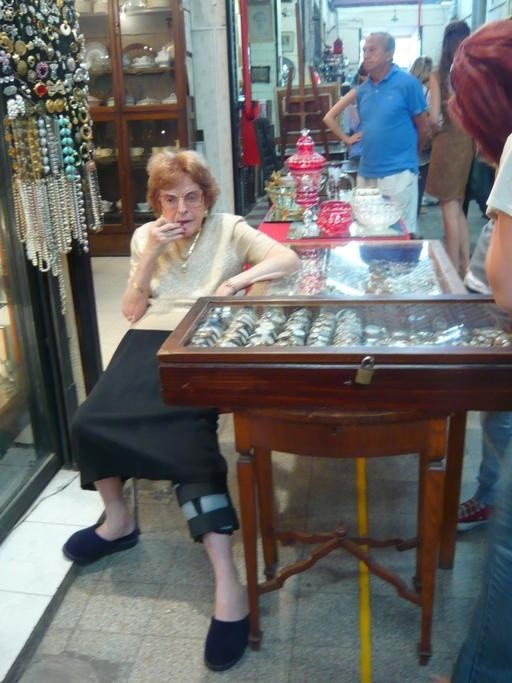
top-left (285, 136), bottom-right (326, 207)
top-left (467, 211), bottom-right (497, 297)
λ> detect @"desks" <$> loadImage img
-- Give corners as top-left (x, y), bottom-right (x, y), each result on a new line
top-left (227, 395), bottom-right (464, 667)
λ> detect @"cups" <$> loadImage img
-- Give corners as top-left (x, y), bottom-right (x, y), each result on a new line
top-left (136, 203), bottom-right (150, 210)
top-left (102, 199), bottom-right (113, 210)
top-left (129, 147), bottom-right (145, 158)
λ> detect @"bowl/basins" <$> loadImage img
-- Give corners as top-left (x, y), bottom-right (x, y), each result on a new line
top-left (264, 185), bottom-right (299, 214)
top-left (354, 202), bottom-right (407, 231)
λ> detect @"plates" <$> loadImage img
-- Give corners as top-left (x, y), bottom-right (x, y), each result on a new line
top-left (86, 42), bottom-right (108, 63)
top-left (134, 210), bottom-right (154, 213)
top-left (121, 42), bottom-right (154, 69)
top-left (102, 209), bottom-right (113, 213)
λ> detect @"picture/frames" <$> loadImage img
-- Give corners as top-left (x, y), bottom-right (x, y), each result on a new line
top-left (251, 65), bottom-right (270, 83)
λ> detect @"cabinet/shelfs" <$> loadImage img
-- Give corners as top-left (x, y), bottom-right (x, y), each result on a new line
top-left (69, 1), bottom-right (200, 257)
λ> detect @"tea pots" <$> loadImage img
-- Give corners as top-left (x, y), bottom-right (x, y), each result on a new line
top-left (155, 46), bottom-right (172, 68)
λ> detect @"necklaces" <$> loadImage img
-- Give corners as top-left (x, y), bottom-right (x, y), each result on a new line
top-left (171, 227), bottom-right (201, 269)
top-left (0, 109), bottom-right (108, 320)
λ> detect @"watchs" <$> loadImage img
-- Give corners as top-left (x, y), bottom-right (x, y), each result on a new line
top-left (186, 299), bottom-right (512, 358)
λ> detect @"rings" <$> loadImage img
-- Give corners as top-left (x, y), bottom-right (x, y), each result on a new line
top-left (166, 231), bottom-right (172, 237)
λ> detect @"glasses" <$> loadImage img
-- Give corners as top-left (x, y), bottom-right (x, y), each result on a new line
top-left (158, 189), bottom-right (204, 209)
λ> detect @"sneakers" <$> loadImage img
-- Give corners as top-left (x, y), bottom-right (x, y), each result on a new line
top-left (62, 522), bottom-right (139, 561)
top-left (456, 495), bottom-right (495, 533)
top-left (204, 615), bottom-right (250, 672)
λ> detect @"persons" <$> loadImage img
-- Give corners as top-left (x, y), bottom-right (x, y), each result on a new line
top-left (325, 63), bottom-right (368, 161)
top-left (410, 21), bottom-right (511, 525)
top-left (354, 33), bottom-right (431, 233)
top-left (63, 148), bottom-right (300, 669)
top-left (444, 141), bottom-right (510, 682)
